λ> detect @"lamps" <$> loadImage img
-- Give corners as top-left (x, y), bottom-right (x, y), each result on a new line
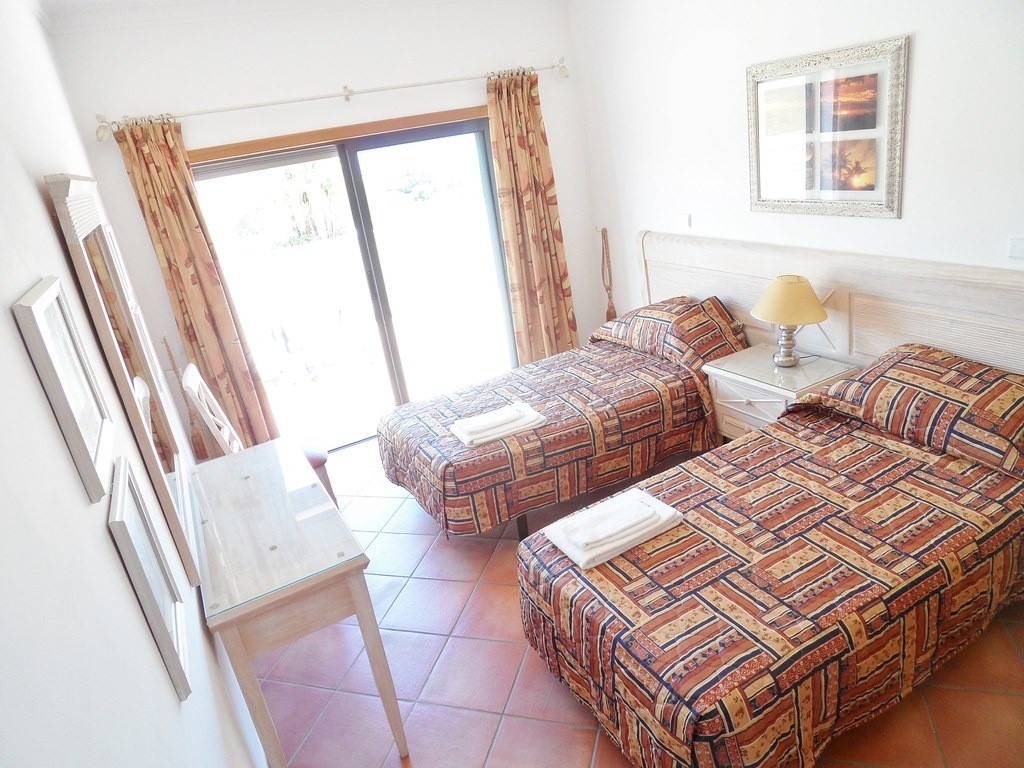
top-left (749, 274), bottom-right (828, 367)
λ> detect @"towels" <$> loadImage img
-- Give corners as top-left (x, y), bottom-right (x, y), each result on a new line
top-left (449, 400), bottom-right (548, 448)
top-left (542, 487), bottom-right (685, 571)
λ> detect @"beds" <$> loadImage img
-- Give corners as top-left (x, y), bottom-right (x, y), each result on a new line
top-left (377, 295), bottom-right (749, 541)
top-left (515, 341), bottom-right (1024, 768)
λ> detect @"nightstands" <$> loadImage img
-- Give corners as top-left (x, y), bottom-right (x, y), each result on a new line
top-left (712, 344), bottom-right (860, 448)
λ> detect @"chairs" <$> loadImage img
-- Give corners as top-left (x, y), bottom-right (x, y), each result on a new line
top-left (181, 363), bottom-right (340, 509)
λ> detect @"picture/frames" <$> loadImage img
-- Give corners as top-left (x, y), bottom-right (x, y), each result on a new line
top-left (747, 34), bottom-right (910, 218)
top-left (12, 276), bottom-right (115, 504)
top-left (108, 455), bottom-right (192, 702)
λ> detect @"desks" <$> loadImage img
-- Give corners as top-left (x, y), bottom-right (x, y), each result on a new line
top-left (190, 435), bottom-right (409, 768)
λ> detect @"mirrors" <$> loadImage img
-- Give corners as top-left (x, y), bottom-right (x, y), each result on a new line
top-left (44, 172), bottom-right (203, 587)
